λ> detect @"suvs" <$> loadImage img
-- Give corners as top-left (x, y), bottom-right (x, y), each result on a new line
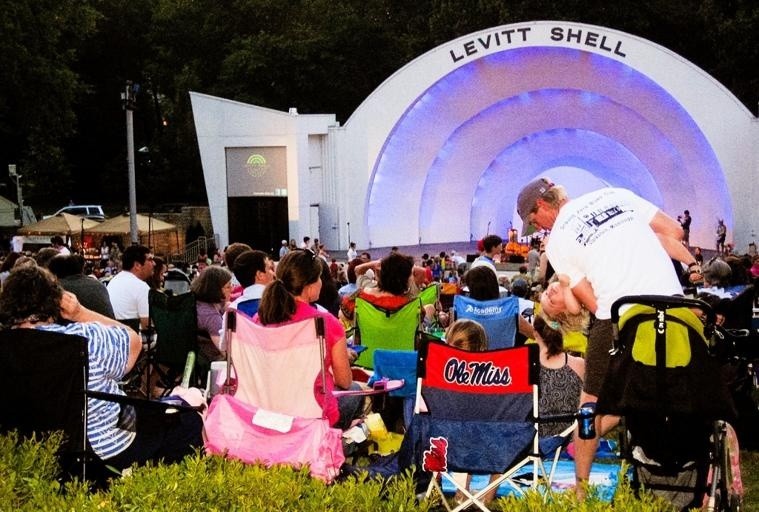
top-left (43, 205), bottom-right (106, 220)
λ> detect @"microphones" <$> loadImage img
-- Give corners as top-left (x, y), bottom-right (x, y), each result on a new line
top-left (488, 221), bottom-right (491, 225)
top-left (508, 221), bottom-right (512, 225)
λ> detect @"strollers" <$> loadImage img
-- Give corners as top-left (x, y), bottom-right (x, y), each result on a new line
top-left (606, 293), bottom-right (743, 512)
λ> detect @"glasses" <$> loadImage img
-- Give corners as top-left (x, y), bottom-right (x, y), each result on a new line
top-left (294, 248), bottom-right (316, 268)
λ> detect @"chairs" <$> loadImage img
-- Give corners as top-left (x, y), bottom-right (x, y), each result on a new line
top-left (452, 295), bottom-right (520, 350)
top-left (407, 333), bottom-right (576, 511)
top-left (348, 294), bottom-right (422, 368)
top-left (148, 292), bottom-right (209, 373)
top-left (420, 285), bottom-right (440, 310)
top-left (2, 331), bottom-right (174, 480)
top-left (224, 308), bottom-right (403, 435)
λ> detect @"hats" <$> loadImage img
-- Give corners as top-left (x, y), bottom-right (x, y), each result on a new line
top-left (517, 178), bottom-right (554, 237)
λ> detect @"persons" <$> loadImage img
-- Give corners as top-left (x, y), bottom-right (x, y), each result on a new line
top-left (251, 250), bottom-right (373, 432)
top-left (28, 208), bottom-right (759, 374)
top-left (532, 316), bottom-right (586, 437)
top-left (518, 179), bottom-right (685, 500)
top-left (0, 266), bottom-right (207, 489)
